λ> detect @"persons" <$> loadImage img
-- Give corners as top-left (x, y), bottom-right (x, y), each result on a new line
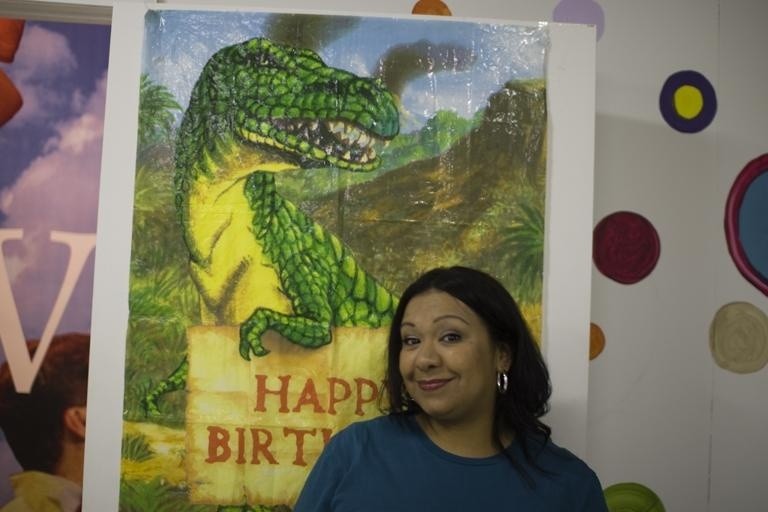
top-left (0, 332), bottom-right (92, 511)
top-left (291, 263), bottom-right (607, 510)
